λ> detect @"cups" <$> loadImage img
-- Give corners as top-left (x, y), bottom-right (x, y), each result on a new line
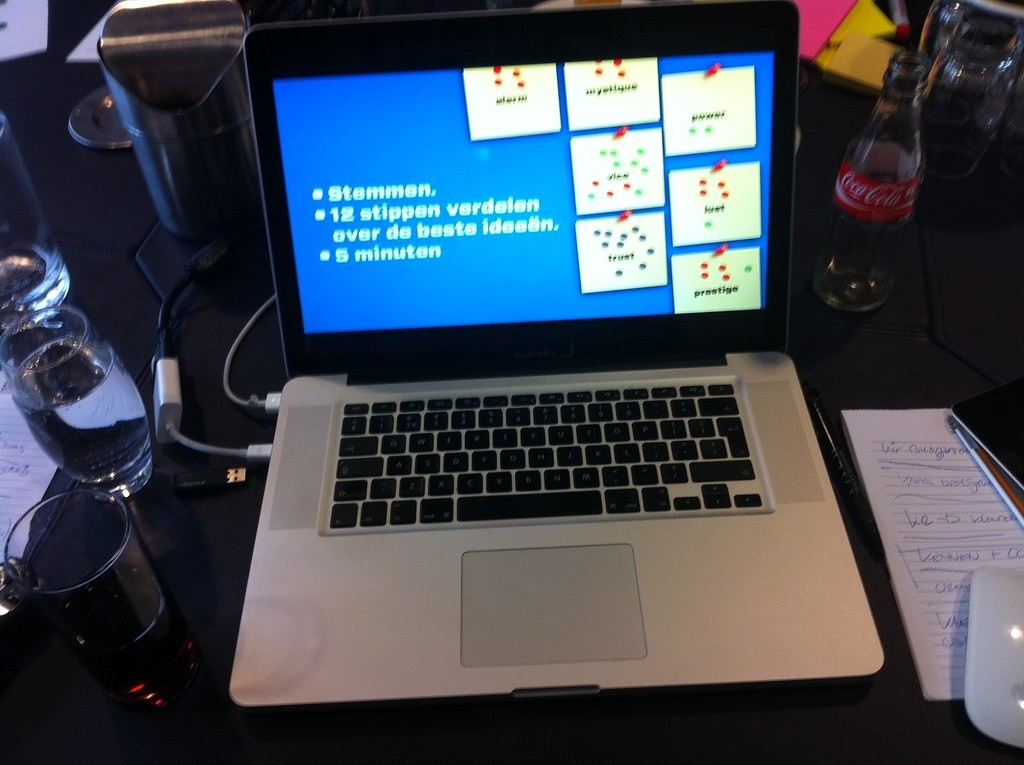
top-left (0, 110), bottom-right (70, 332)
top-left (4, 490), bottom-right (202, 712)
top-left (915, 0), bottom-right (1024, 180)
top-left (0, 306), bottom-right (153, 501)
top-left (96, 0), bottom-right (259, 239)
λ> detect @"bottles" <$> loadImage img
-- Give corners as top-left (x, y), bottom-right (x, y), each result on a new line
top-left (812, 48), bottom-right (933, 314)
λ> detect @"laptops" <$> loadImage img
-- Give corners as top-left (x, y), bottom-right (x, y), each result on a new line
top-left (230, 0), bottom-right (884, 710)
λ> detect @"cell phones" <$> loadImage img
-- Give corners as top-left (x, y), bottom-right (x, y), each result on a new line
top-left (950, 378), bottom-right (1024, 501)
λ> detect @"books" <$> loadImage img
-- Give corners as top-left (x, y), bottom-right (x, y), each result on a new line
top-left (840, 408), bottom-right (1024, 702)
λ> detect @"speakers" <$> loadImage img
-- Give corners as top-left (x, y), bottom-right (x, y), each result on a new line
top-left (95, 0), bottom-right (262, 288)
